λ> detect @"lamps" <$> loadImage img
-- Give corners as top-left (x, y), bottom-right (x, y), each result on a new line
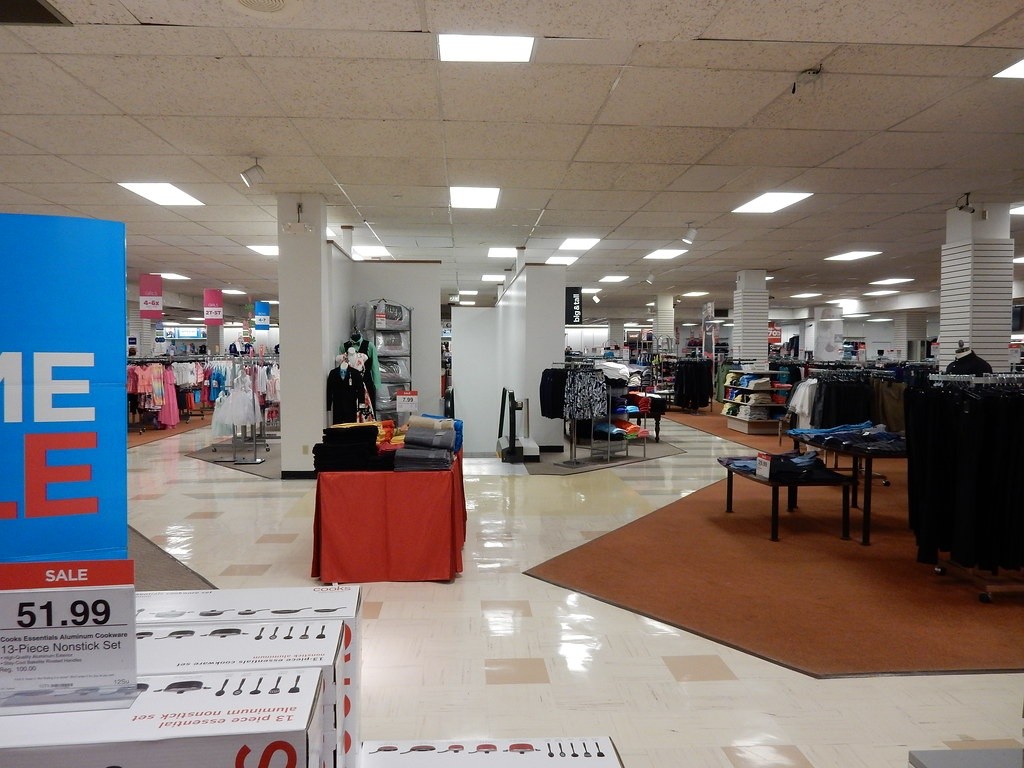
top-left (592, 293), bottom-right (601, 304)
top-left (240, 153), bottom-right (266, 187)
top-left (282, 221), bottom-right (316, 235)
top-left (680, 222), bottom-right (698, 245)
top-left (645, 271), bottom-right (656, 285)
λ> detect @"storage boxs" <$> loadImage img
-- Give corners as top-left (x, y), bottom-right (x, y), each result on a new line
top-left (0, 586), bottom-right (624, 768)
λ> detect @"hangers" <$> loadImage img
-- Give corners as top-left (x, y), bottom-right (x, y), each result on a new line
top-left (772, 357), bottom-right (935, 385)
top-left (928, 372), bottom-right (1024, 393)
top-left (129, 352), bottom-right (279, 372)
top-left (552, 353), bottom-right (713, 376)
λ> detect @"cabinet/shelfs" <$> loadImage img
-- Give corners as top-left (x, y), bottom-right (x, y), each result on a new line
top-left (352, 298), bottom-right (413, 425)
top-left (720, 369), bottom-right (790, 435)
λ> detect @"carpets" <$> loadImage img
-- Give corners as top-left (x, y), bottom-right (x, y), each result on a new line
top-left (128, 408), bottom-right (214, 451)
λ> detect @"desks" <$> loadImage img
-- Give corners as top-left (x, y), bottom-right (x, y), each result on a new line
top-left (785, 429), bottom-right (908, 546)
top-left (718, 451), bottom-right (858, 541)
top-left (311, 447), bottom-right (468, 585)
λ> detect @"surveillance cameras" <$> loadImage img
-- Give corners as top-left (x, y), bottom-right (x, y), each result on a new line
top-left (959, 206), bottom-right (975, 214)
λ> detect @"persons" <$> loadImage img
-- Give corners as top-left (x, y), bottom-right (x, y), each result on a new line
top-left (327, 362), bottom-right (365, 424)
top-left (944, 346), bottom-right (994, 377)
top-left (336, 347), bottom-right (377, 423)
top-left (339, 334), bottom-right (382, 422)
